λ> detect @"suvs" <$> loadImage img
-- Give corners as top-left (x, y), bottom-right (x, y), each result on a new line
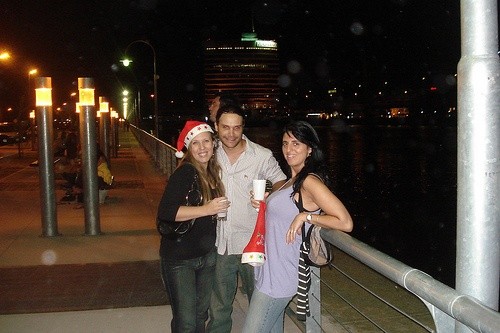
top-left (0, 122), bottom-right (26, 146)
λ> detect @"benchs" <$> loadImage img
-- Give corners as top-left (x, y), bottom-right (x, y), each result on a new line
top-left (99, 176), bottom-right (114, 204)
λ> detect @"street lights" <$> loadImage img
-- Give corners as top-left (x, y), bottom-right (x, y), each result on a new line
top-left (123, 97), bottom-right (138, 135)
top-left (120, 38), bottom-right (160, 169)
top-left (122, 74), bottom-right (142, 153)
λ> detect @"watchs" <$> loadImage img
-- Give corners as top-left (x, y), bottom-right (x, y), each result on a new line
top-left (306, 212), bottom-right (312, 223)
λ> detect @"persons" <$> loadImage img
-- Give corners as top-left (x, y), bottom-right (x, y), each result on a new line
top-left (123, 119), bottom-right (130, 132)
top-left (156, 119), bottom-right (229, 333)
top-left (60, 144), bottom-right (114, 205)
top-left (241, 123), bottom-right (353, 333)
top-left (211, 106), bottom-right (287, 333)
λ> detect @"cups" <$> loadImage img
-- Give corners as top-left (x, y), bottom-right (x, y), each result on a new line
top-left (216, 197), bottom-right (228, 218)
top-left (252, 179), bottom-right (266, 201)
